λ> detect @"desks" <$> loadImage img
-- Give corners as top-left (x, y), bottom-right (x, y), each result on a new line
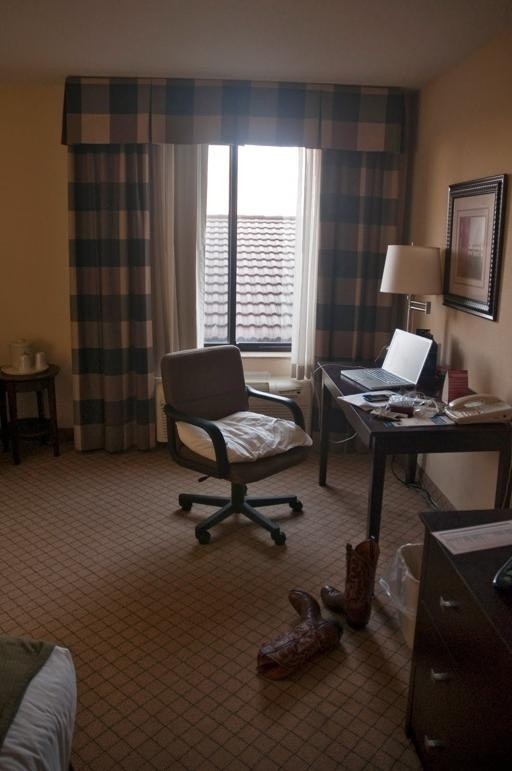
top-left (0, 364), bottom-right (60, 466)
top-left (315, 360), bottom-right (511, 545)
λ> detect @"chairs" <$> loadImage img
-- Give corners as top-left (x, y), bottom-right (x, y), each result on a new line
top-left (160, 345), bottom-right (309, 545)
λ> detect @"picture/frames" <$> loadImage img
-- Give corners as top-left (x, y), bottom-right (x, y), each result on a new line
top-left (442, 173), bottom-right (507, 322)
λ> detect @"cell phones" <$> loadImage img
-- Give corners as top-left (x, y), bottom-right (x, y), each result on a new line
top-left (362, 394), bottom-right (397, 403)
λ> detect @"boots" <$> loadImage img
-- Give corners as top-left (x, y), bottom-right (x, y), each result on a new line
top-left (256, 586), bottom-right (344, 680)
top-left (322, 537), bottom-right (380, 631)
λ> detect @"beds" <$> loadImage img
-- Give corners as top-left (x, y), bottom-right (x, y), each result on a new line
top-left (1, 638), bottom-right (78, 770)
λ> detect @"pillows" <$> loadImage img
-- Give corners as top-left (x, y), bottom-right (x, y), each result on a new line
top-left (174, 411), bottom-right (313, 464)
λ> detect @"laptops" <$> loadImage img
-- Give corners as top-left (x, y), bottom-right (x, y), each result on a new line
top-left (340, 329), bottom-right (434, 391)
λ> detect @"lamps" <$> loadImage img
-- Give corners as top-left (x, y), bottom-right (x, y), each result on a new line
top-left (380, 245), bottom-right (442, 335)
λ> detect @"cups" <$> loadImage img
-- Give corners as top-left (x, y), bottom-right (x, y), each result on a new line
top-left (35, 352), bottom-right (46, 369)
top-left (19, 355), bottom-right (31, 373)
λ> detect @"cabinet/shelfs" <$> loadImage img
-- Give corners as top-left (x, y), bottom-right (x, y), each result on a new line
top-left (404, 509), bottom-right (512, 770)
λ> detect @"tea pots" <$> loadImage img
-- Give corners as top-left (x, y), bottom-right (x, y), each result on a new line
top-left (8, 339), bottom-right (31, 368)
